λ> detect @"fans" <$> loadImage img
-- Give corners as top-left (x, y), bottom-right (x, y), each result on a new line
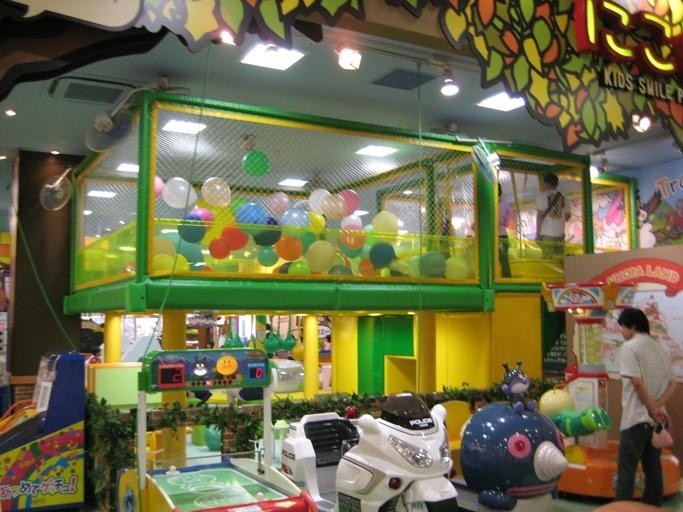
top-left (82, 81), bottom-right (150, 160)
top-left (39, 164), bottom-right (76, 211)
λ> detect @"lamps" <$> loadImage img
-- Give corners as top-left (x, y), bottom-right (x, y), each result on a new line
top-left (440, 68), bottom-right (460, 96)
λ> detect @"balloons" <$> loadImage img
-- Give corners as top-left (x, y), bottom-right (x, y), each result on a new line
top-left (153, 176), bottom-right (474, 281)
top-left (241, 150), bottom-right (272, 176)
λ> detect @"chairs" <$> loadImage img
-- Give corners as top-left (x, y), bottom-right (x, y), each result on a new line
top-left (302, 416), bottom-right (361, 468)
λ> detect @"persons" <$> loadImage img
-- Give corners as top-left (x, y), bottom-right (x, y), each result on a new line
top-left (498, 183), bottom-right (512, 278)
top-left (536, 173), bottom-right (571, 254)
top-left (616, 308), bottom-right (677, 507)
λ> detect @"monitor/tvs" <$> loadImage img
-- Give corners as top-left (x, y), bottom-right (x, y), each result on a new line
top-left (36, 384), bottom-right (49, 410)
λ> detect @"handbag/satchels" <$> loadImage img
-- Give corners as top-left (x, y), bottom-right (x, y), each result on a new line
top-left (651, 429), bottom-right (674, 449)
